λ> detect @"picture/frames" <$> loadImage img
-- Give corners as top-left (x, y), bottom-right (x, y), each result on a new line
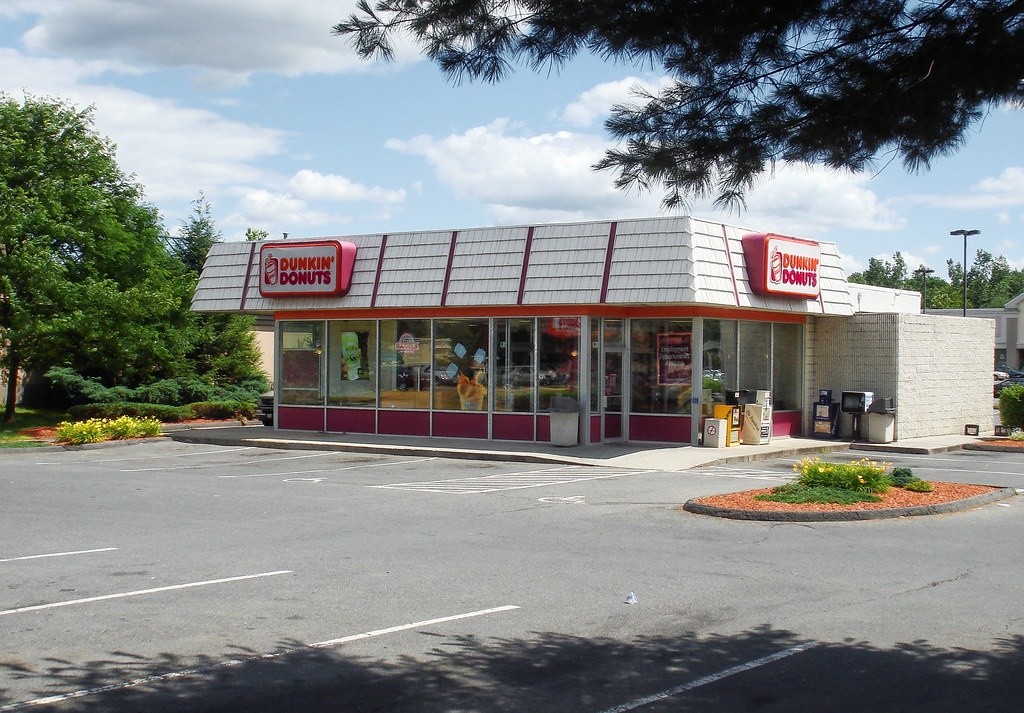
top-left (656, 333), bottom-right (692, 385)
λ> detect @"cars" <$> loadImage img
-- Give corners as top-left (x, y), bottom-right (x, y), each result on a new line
top-left (395, 362), bottom-right (557, 387)
top-left (994, 367), bottom-right (1024, 398)
top-left (257, 381), bottom-right (274, 426)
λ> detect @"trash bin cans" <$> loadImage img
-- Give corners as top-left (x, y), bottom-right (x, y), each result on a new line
top-left (548, 396), bottom-right (580, 446)
top-left (868, 397), bottom-right (896, 443)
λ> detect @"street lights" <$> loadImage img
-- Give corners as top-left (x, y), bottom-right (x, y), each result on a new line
top-left (915, 268), bottom-right (935, 314)
top-left (950, 229), bottom-right (981, 317)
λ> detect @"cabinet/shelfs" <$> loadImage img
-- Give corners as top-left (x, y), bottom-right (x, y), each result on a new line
top-left (714, 405), bottom-right (740, 447)
top-left (743, 403), bottom-right (774, 444)
top-left (813, 402), bottom-right (840, 439)
